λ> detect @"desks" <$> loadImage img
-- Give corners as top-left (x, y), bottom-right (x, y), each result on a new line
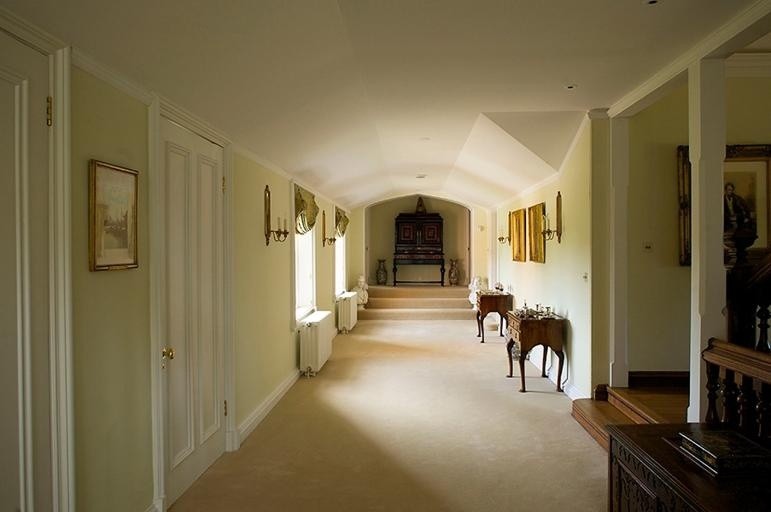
top-left (506, 309), bottom-right (565, 392)
top-left (476, 292), bottom-right (510, 343)
top-left (607, 423), bottom-right (771, 512)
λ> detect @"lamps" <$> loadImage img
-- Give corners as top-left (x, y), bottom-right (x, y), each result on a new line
top-left (536, 191), bottom-right (562, 242)
top-left (322, 210), bottom-right (336, 247)
top-left (497, 211), bottom-right (511, 246)
top-left (264, 183), bottom-right (289, 245)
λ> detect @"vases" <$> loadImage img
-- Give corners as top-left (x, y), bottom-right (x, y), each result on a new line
top-left (375, 259), bottom-right (388, 285)
top-left (449, 258), bottom-right (459, 287)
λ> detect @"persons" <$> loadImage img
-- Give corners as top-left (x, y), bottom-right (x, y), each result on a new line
top-left (724, 182), bottom-right (753, 232)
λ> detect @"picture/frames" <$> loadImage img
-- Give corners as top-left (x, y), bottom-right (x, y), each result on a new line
top-left (676, 143), bottom-right (771, 266)
top-left (86, 159), bottom-right (139, 272)
top-left (511, 208), bottom-right (526, 262)
top-left (528, 202), bottom-right (546, 264)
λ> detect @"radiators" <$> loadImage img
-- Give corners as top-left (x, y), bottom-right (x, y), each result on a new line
top-left (337, 291), bottom-right (357, 334)
top-left (298, 309), bottom-right (334, 375)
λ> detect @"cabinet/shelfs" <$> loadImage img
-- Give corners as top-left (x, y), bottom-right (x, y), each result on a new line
top-left (393, 212), bottom-right (444, 286)
